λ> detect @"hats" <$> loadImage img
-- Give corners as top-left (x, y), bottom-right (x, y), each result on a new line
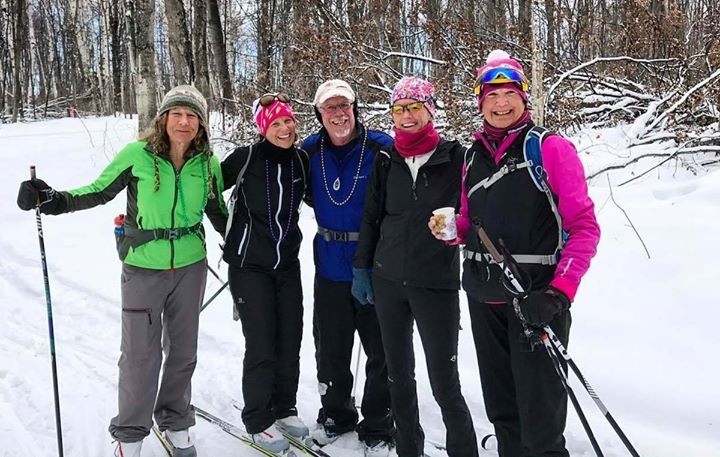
top-left (390, 76), bottom-right (436, 120)
top-left (473, 50), bottom-right (529, 112)
top-left (158, 85), bottom-right (207, 124)
top-left (252, 92), bottom-right (295, 137)
top-left (314, 79), bottom-right (355, 106)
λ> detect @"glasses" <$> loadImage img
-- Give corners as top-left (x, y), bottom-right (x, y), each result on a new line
top-left (473, 67), bottom-right (529, 90)
top-left (255, 93), bottom-right (291, 115)
top-left (388, 96), bottom-right (433, 115)
top-left (319, 102), bottom-right (354, 112)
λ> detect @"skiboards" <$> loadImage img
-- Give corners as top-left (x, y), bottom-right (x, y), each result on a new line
top-left (184, 393), bottom-right (332, 456)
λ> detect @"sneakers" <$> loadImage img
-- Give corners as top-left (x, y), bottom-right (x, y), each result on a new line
top-left (363, 438), bottom-right (389, 457)
top-left (275, 407), bottom-right (309, 436)
top-left (250, 423), bottom-right (290, 453)
top-left (165, 429), bottom-right (197, 457)
top-left (312, 423), bottom-right (340, 446)
top-left (118, 440), bottom-right (143, 457)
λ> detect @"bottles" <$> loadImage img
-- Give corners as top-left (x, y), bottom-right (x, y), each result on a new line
top-left (113, 214), bottom-right (126, 250)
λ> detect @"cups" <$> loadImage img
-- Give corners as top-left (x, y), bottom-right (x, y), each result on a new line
top-left (432, 206), bottom-right (457, 241)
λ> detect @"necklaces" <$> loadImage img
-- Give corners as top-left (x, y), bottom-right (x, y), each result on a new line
top-left (265, 160), bottom-right (293, 241)
top-left (321, 126), bottom-right (367, 205)
top-left (173, 155), bottom-right (206, 235)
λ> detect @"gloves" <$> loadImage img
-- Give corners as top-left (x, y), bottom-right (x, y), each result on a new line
top-left (351, 266), bottom-right (375, 305)
top-left (17, 178), bottom-right (61, 215)
top-left (520, 287), bottom-right (571, 326)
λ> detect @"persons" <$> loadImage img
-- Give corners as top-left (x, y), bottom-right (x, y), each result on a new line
top-left (428, 50), bottom-right (602, 457)
top-left (17, 85), bottom-right (230, 457)
top-left (204, 93), bottom-right (315, 451)
top-left (352, 77), bottom-right (469, 457)
top-left (299, 78), bottom-right (394, 457)
